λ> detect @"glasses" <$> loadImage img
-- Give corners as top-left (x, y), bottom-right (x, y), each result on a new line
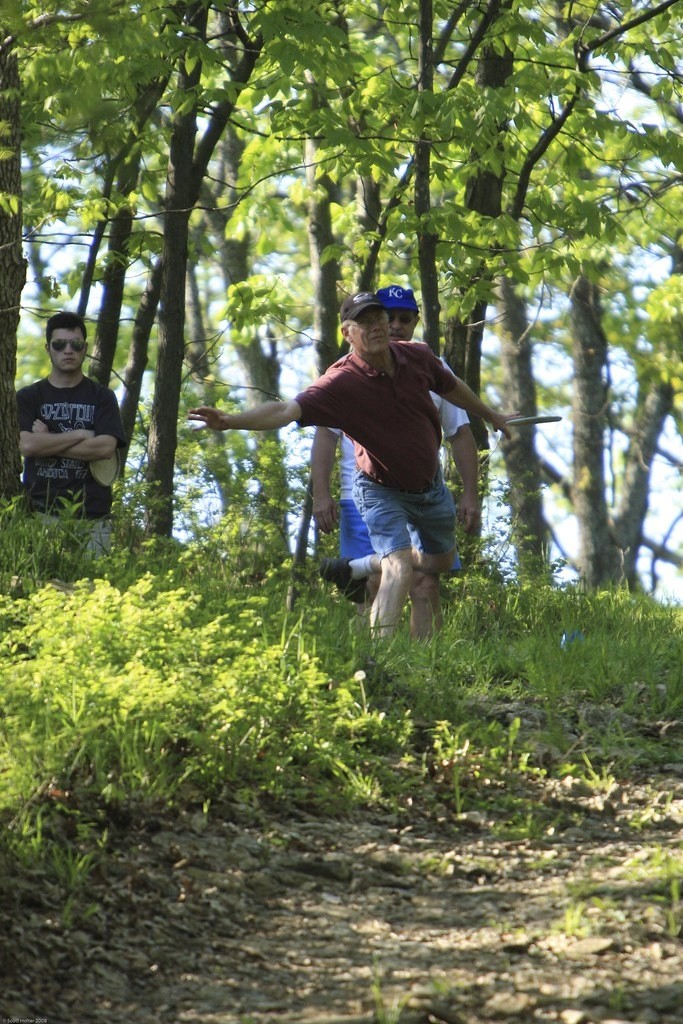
top-left (49, 337), bottom-right (85, 353)
top-left (348, 313), bottom-right (391, 330)
top-left (386, 313), bottom-right (416, 324)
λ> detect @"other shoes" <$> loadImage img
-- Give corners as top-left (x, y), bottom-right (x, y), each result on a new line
top-left (319, 558), bottom-right (370, 604)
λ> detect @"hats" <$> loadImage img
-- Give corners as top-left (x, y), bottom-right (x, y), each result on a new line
top-left (339, 292), bottom-right (382, 323)
top-left (375, 285), bottom-right (420, 315)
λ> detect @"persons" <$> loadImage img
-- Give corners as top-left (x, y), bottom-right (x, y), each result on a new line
top-left (187, 292), bottom-right (526, 649)
top-left (15, 312), bottom-right (126, 563)
top-left (311, 285), bottom-right (483, 641)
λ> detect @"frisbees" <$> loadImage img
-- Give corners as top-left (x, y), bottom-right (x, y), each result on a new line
top-left (505, 416), bottom-right (562, 426)
top-left (89, 448), bottom-right (121, 487)
